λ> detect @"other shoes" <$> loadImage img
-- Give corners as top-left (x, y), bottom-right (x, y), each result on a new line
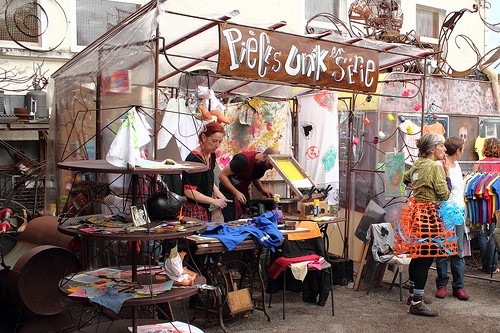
top-left (409, 302), bottom-right (438, 315)
top-left (490, 268), bottom-right (500, 273)
top-left (436, 288), bottom-right (447, 298)
top-left (453, 288), bottom-right (469, 300)
top-left (406, 295), bottom-right (432, 305)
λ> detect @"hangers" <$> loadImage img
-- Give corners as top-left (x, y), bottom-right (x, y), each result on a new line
top-left (459, 158), bottom-right (500, 177)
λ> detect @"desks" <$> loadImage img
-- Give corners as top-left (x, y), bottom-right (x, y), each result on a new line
top-left (188, 240), bottom-right (271, 333)
top-left (318, 218), bottom-right (347, 241)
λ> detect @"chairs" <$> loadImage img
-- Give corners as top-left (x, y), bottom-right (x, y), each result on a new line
top-left (491, 210), bottom-right (500, 282)
top-left (366, 222), bottom-right (411, 301)
top-left (278, 234), bottom-right (334, 317)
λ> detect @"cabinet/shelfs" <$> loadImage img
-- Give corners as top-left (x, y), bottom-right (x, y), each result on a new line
top-left (58, 162), bottom-right (209, 333)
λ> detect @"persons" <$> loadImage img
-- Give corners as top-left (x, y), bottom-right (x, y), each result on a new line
top-left (435, 135), bottom-right (473, 299)
top-left (403, 132), bottom-right (452, 317)
top-left (218, 147), bottom-right (282, 274)
top-left (179, 123), bottom-right (233, 309)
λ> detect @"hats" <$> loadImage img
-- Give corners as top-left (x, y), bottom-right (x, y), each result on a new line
top-left (206, 121), bottom-right (224, 132)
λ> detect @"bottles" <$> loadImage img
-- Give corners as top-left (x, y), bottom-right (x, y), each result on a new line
top-left (314, 199), bottom-right (320, 217)
